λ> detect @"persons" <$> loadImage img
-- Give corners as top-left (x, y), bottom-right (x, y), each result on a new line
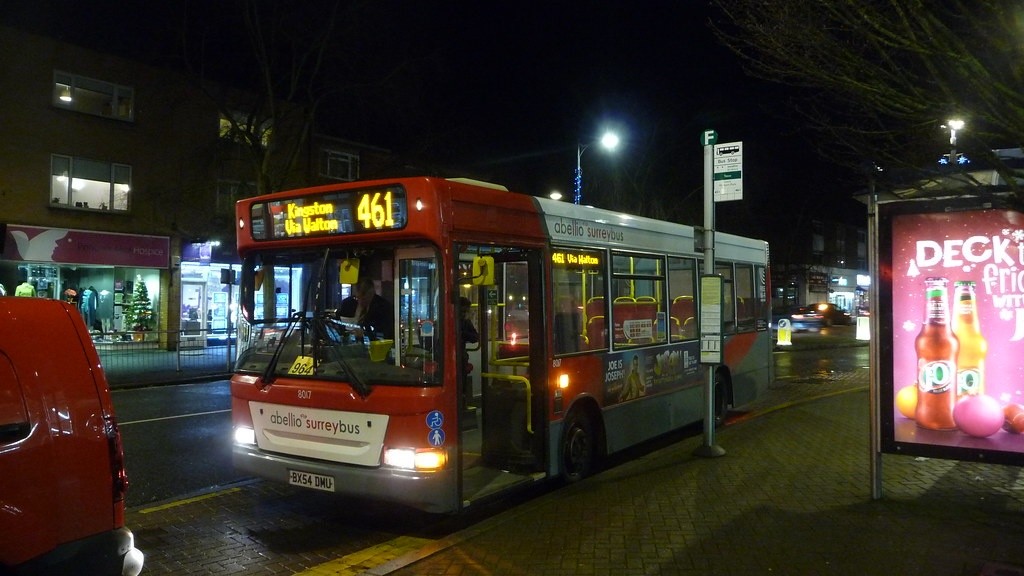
top-left (78, 289), bottom-right (97, 330)
top-left (15, 283), bottom-right (35, 298)
top-left (618, 355), bottom-right (645, 403)
top-left (432, 297), bottom-right (478, 389)
top-left (555, 301), bottom-right (581, 352)
top-left (0, 284), bottom-right (7, 297)
top-left (335, 277), bottom-right (397, 344)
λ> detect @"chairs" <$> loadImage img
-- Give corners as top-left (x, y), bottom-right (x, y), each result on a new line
top-left (493, 295), bottom-right (742, 358)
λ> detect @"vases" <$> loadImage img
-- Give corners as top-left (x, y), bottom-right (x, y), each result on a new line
top-left (70, 298), bottom-right (72, 302)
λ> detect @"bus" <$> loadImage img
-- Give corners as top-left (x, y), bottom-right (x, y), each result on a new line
top-left (229, 177), bottom-right (775, 523)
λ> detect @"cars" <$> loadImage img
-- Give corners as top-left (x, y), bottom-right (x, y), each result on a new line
top-left (771, 303), bottom-right (852, 332)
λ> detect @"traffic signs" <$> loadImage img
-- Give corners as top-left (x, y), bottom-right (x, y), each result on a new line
top-left (714, 142), bottom-right (743, 202)
top-left (776, 319), bottom-right (792, 346)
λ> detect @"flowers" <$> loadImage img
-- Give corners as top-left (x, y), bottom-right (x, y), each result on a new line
top-left (64, 289), bottom-right (76, 298)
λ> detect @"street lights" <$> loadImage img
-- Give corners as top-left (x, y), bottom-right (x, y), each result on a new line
top-left (572, 126), bottom-right (621, 206)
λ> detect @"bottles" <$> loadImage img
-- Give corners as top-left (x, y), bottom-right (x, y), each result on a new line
top-left (950, 280), bottom-right (988, 402)
top-left (914, 277), bottom-right (960, 431)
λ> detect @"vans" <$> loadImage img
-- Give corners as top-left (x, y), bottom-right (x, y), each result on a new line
top-left (0, 297), bottom-right (144, 576)
